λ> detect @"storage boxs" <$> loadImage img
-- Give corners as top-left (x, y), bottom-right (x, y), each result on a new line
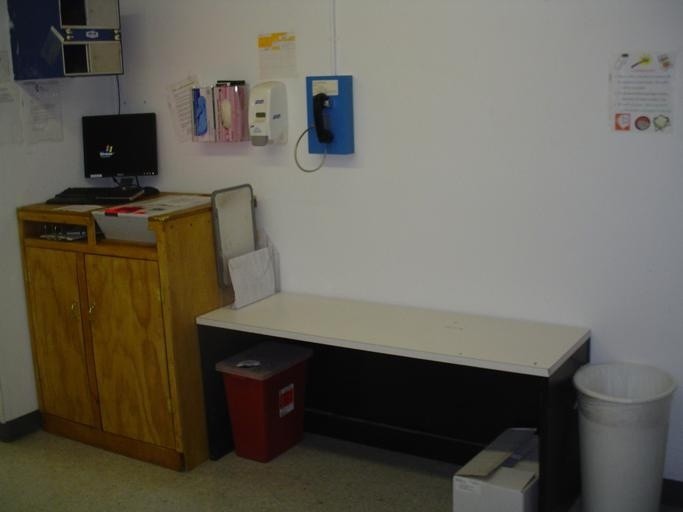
top-left (451, 425), bottom-right (541, 512)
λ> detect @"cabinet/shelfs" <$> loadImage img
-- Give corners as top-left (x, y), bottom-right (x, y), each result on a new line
top-left (18, 189), bottom-right (257, 475)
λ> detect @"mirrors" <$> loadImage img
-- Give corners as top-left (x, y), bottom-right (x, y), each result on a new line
top-left (211, 183), bottom-right (260, 291)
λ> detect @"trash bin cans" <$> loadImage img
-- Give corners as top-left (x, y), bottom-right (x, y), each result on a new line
top-left (574, 362), bottom-right (677, 512)
top-left (216, 341), bottom-right (313, 463)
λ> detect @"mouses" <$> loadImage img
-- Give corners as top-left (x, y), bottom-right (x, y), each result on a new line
top-left (144, 186), bottom-right (159, 194)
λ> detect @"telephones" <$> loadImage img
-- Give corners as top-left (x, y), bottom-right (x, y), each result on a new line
top-left (313, 93), bottom-right (334, 143)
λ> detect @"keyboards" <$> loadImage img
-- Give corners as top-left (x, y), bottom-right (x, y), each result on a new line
top-left (46, 184), bottom-right (146, 202)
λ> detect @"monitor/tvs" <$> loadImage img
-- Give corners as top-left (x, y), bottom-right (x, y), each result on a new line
top-left (82, 112), bottom-right (158, 184)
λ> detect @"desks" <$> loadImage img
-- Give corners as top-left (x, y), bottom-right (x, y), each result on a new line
top-left (194, 293), bottom-right (590, 512)
